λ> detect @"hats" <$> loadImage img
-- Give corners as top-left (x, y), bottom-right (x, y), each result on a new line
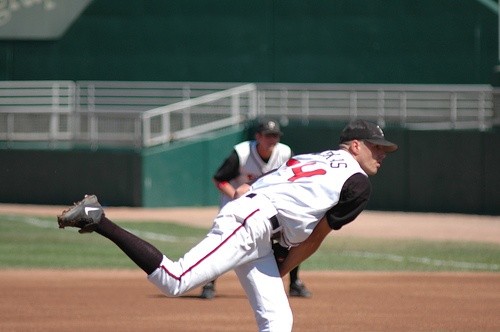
top-left (258, 118), bottom-right (284, 136)
top-left (341, 119), bottom-right (397, 153)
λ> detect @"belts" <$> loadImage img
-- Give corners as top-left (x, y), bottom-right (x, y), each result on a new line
top-left (246, 193), bottom-right (282, 240)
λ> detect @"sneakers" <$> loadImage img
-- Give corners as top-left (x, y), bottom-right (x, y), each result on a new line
top-left (57, 194), bottom-right (104, 233)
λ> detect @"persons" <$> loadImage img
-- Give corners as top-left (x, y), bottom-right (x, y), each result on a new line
top-left (198, 120), bottom-right (313, 298)
top-left (57, 119), bottom-right (399, 332)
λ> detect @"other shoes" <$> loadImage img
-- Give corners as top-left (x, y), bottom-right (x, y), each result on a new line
top-left (201, 285), bottom-right (216, 299)
top-left (290, 281), bottom-right (310, 297)
